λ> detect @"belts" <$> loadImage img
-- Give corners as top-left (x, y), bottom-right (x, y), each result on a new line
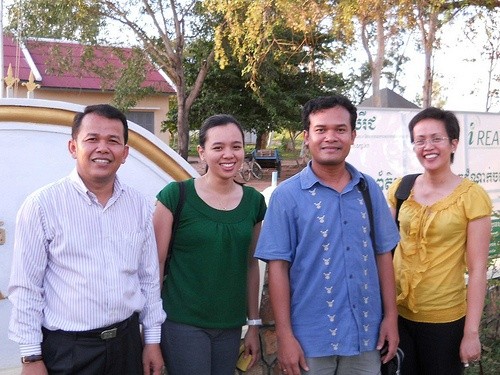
top-left (53, 312), bottom-right (139, 340)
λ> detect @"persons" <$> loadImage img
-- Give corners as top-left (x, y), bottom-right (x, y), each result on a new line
top-left (6, 104), bottom-right (168, 375)
top-left (253, 96), bottom-right (402, 375)
top-left (153, 114), bottom-right (267, 375)
top-left (380, 107), bottom-right (492, 375)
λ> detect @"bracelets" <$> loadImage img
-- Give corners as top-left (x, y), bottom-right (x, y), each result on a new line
top-left (21, 355), bottom-right (42, 364)
top-left (247, 319), bottom-right (262, 325)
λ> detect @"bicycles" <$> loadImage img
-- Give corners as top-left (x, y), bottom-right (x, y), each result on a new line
top-left (240, 147), bottom-right (264, 182)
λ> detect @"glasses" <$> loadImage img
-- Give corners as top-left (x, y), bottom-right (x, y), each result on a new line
top-left (411, 136), bottom-right (452, 149)
